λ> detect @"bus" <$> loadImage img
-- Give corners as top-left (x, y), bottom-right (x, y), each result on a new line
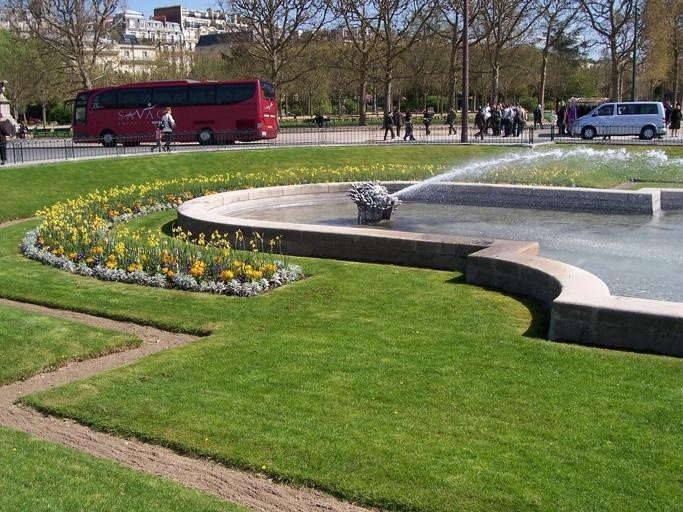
top-left (62, 79), bottom-right (280, 144)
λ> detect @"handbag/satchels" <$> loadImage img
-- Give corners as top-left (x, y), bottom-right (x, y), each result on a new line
top-left (169, 119), bottom-right (177, 128)
top-left (159, 120), bottom-right (165, 130)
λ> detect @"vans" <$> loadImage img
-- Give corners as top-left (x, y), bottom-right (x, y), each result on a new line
top-left (571, 101), bottom-right (666, 139)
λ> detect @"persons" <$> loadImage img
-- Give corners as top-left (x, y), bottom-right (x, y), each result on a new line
top-left (665, 100), bottom-right (683, 137)
top-left (159, 106), bottom-right (177, 152)
top-left (395, 98), bottom-right (578, 141)
top-left (384, 110), bottom-right (396, 140)
top-left (315, 111), bottom-right (324, 128)
top-left (0, 110), bottom-right (14, 165)
top-left (150, 126), bottom-right (163, 152)
